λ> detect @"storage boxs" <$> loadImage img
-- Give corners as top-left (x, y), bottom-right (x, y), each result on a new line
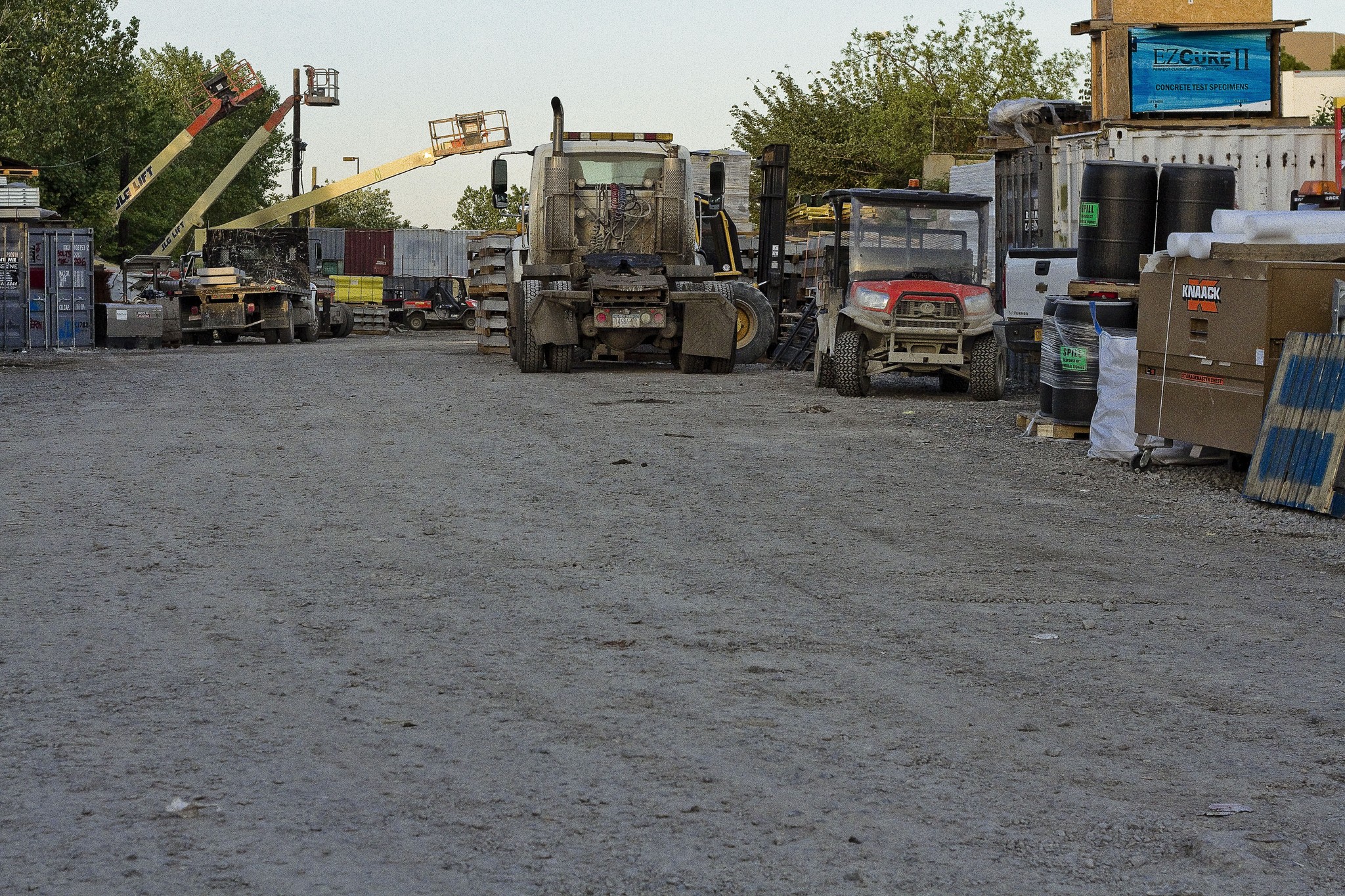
top-left (313, 228), bottom-right (487, 300)
top-left (1051, 128), bottom-right (1335, 253)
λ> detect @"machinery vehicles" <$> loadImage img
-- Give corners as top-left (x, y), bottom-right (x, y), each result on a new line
top-left (491, 96), bottom-right (777, 375)
top-left (93, 58), bottom-right (513, 345)
top-left (804, 177), bottom-right (1010, 402)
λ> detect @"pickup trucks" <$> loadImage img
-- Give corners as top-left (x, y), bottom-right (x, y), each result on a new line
top-left (1001, 180), bottom-right (1345, 354)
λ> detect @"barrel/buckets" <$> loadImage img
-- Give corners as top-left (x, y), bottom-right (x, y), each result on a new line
top-left (1039, 294), bottom-right (1138, 424)
top-left (1077, 159), bottom-right (1159, 285)
top-left (1154, 162), bottom-right (1238, 251)
